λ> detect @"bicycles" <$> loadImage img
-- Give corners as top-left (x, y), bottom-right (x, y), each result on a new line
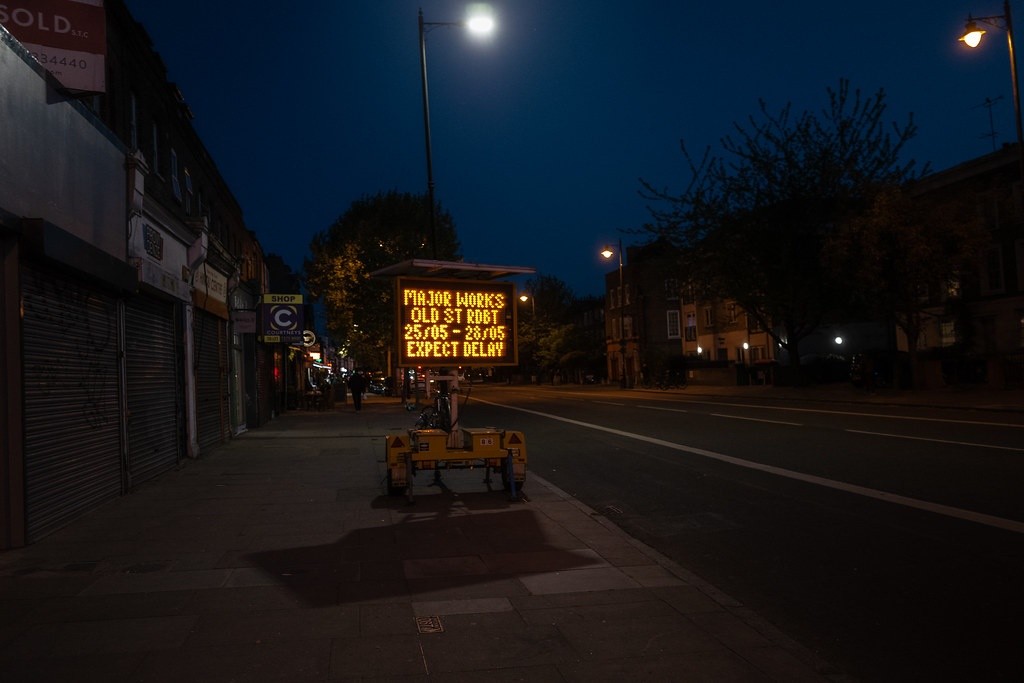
top-left (405, 400), bottom-right (439, 441)
top-left (369, 378), bottom-right (396, 397)
top-left (640, 361), bottom-right (689, 391)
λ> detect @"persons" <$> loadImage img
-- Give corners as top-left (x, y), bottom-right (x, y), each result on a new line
top-left (313, 379), bottom-right (349, 410)
top-left (346, 367), bottom-right (366, 414)
top-left (639, 361), bottom-right (649, 386)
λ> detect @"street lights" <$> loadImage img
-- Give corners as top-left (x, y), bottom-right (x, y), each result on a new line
top-left (600, 238), bottom-right (625, 379)
top-left (958, 1), bottom-right (1024, 150)
top-left (419, 3), bottom-right (502, 257)
top-left (520, 292), bottom-right (537, 337)
